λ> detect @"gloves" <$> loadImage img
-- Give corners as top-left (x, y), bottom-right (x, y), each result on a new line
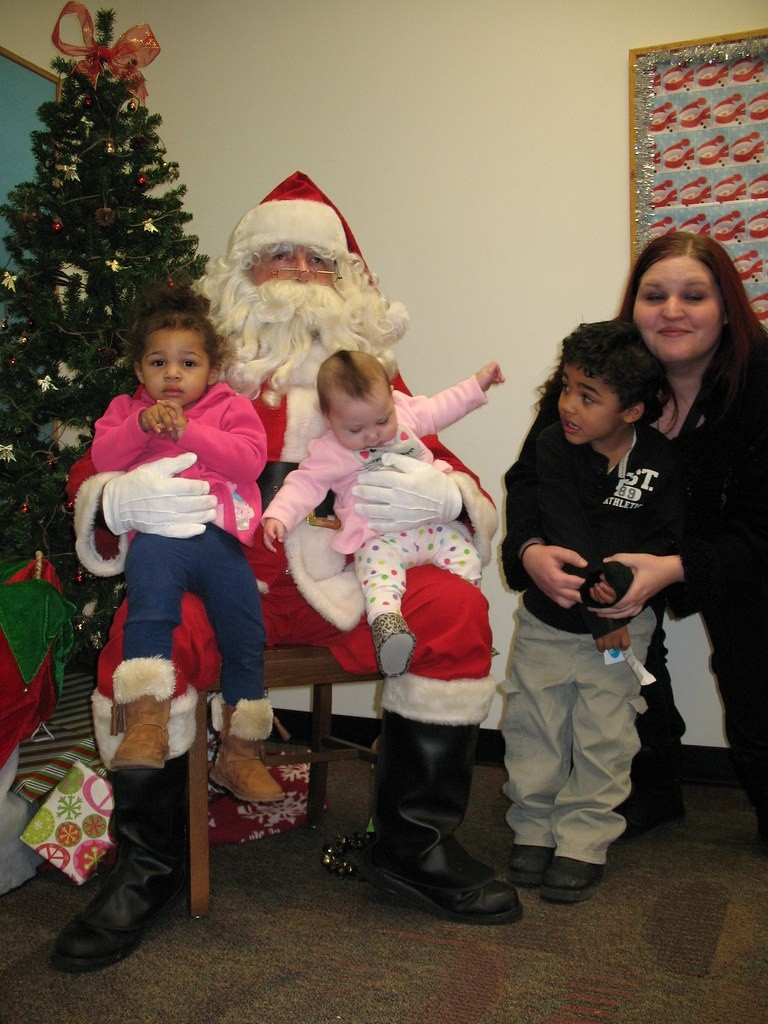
top-left (101, 452), bottom-right (217, 539)
top-left (350, 452), bottom-right (462, 533)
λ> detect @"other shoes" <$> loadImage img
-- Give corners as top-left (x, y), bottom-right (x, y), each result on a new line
top-left (611, 787), bottom-right (685, 840)
top-left (508, 844), bottom-right (553, 885)
top-left (541, 854), bottom-right (602, 902)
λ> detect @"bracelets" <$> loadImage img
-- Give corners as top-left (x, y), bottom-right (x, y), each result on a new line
top-left (520, 542), bottom-right (540, 564)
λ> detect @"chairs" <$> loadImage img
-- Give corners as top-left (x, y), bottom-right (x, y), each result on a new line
top-left (185, 645), bottom-right (386, 925)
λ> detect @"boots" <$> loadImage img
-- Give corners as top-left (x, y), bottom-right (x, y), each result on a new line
top-left (209, 698), bottom-right (285, 802)
top-left (52, 687), bottom-right (199, 971)
top-left (110, 656), bottom-right (175, 770)
top-left (358, 671), bottom-right (523, 923)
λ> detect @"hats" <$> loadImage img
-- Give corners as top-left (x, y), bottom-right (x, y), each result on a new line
top-left (226, 172), bottom-right (411, 344)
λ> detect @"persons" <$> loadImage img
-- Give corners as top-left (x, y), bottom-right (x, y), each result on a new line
top-left (501, 321), bottom-right (683, 902)
top-left (89, 285), bottom-right (286, 803)
top-left (503, 231), bottom-right (767, 842)
top-left (54, 169), bottom-right (525, 973)
top-left (260, 349), bottom-right (507, 678)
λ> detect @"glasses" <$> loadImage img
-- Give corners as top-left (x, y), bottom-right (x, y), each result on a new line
top-left (260, 257), bottom-right (342, 285)
